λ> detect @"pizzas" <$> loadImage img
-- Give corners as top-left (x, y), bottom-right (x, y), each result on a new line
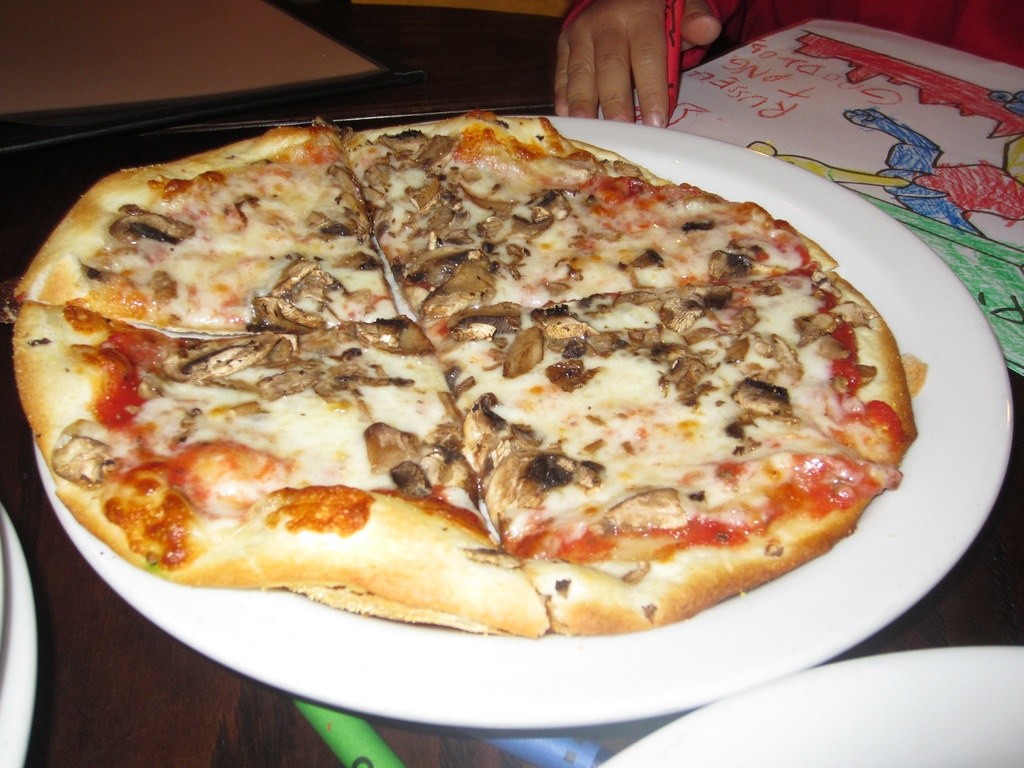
top-left (10, 109), bottom-right (920, 639)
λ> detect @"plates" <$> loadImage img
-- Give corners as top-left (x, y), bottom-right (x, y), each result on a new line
top-left (30, 116), bottom-right (1013, 730)
top-left (595, 646), bottom-right (1024, 768)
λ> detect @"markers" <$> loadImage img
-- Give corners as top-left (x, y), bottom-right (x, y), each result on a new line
top-left (293, 697), bottom-right (617, 768)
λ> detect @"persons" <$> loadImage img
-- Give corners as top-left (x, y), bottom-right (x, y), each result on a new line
top-left (555, 0), bottom-right (1024, 129)
